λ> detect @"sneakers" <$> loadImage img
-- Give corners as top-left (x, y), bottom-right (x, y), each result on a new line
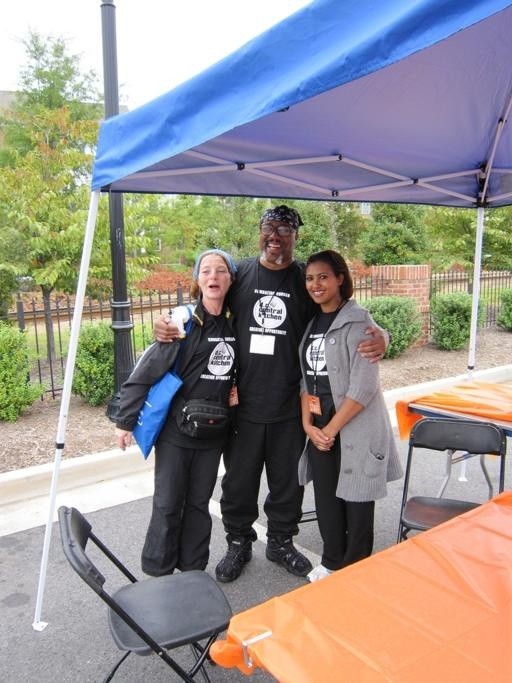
top-left (215, 534), bottom-right (252, 583)
top-left (266, 535), bottom-right (335, 583)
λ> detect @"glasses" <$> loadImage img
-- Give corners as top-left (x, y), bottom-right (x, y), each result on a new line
top-left (260, 223), bottom-right (297, 236)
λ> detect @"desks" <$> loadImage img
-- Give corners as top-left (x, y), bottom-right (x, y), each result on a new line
top-left (394, 380), bottom-right (512, 501)
top-left (209, 491), bottom-right (512, 683)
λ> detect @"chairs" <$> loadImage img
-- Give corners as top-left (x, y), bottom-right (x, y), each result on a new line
top-left (57, 505), bottom-right (233, 683)
top-left (395, 417), bottom-right (506, 544)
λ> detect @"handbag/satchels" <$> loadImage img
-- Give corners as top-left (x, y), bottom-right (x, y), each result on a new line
top-left (175, 398), bottom-right (231, 441)
top-left (132, 371), bottom-right (183, 461)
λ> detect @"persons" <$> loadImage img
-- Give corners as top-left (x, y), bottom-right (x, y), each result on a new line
top-left (115, 249), bottom-right (240, 577)
top-left (153, 204), bottom-right (388, 583)
top-left (298, 250), bottom-right (403, 583)
